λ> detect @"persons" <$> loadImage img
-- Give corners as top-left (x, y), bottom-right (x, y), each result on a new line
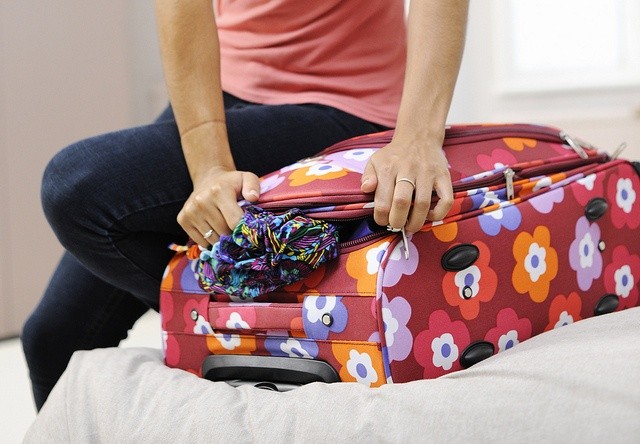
top-left (22, 0), bottom-right (470, 414)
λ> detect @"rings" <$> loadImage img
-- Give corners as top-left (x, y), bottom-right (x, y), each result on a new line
top-left (396, 178), bottom-right (415, 189)
top-left (204, 229), bottom-right (214, 239)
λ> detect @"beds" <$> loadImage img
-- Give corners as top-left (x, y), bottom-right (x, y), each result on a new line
top-left (26, 88), bottom-right (640, 444)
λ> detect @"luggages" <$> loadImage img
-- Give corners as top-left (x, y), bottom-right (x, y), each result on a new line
top-left (160, 124), bottom-right (640, 392)
